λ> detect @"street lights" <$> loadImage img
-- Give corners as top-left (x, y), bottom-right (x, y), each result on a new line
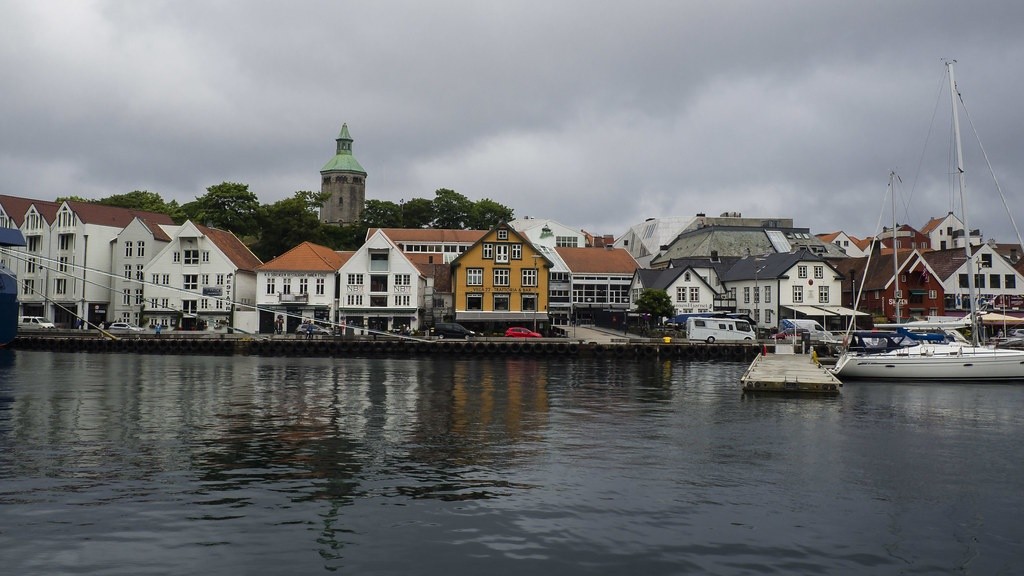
top-left (754, 265), bottom-right (768, 338)
top-left (531, 254), bottom-right (543, 333)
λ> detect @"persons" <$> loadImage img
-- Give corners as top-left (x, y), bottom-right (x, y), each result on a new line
top-left (153, 323), bottom-right (161, 339)
top-left (98, 321), bottom-right (104, 338)
top-left (623, 321), bottom-right (664, 338)
top-left (76, 317), bottom-right (85, 329)
top-left (998, 329), bottom-right (1004, 338)
top-left (272, 317), bottom-right (411, 340)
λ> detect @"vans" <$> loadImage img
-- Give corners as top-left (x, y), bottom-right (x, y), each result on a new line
top-left (782, 319), bottom-right (834, 337)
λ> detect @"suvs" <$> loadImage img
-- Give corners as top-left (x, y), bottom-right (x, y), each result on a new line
top-left (18, 315), bottom-right (55, 329)
top-left (435, 322), bottom-right (479, 339)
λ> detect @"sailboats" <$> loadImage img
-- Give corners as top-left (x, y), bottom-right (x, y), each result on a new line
top-left (818, 57), bottom-right (1024, 381)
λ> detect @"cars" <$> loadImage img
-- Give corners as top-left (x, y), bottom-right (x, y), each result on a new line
top-left (295, 324), bottom-right (332, 337)
top-left (504, 328), bottom-right (542, 339)
top-left (1007, 329), bottom-right (1024, 338)
top-left (768, 327), bottom-right (810, 340)
top-left (108, 321), bottom-right (146, 331)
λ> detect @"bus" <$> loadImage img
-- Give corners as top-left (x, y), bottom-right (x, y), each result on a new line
top-left (686, 316), bottom-right (756, 344)
